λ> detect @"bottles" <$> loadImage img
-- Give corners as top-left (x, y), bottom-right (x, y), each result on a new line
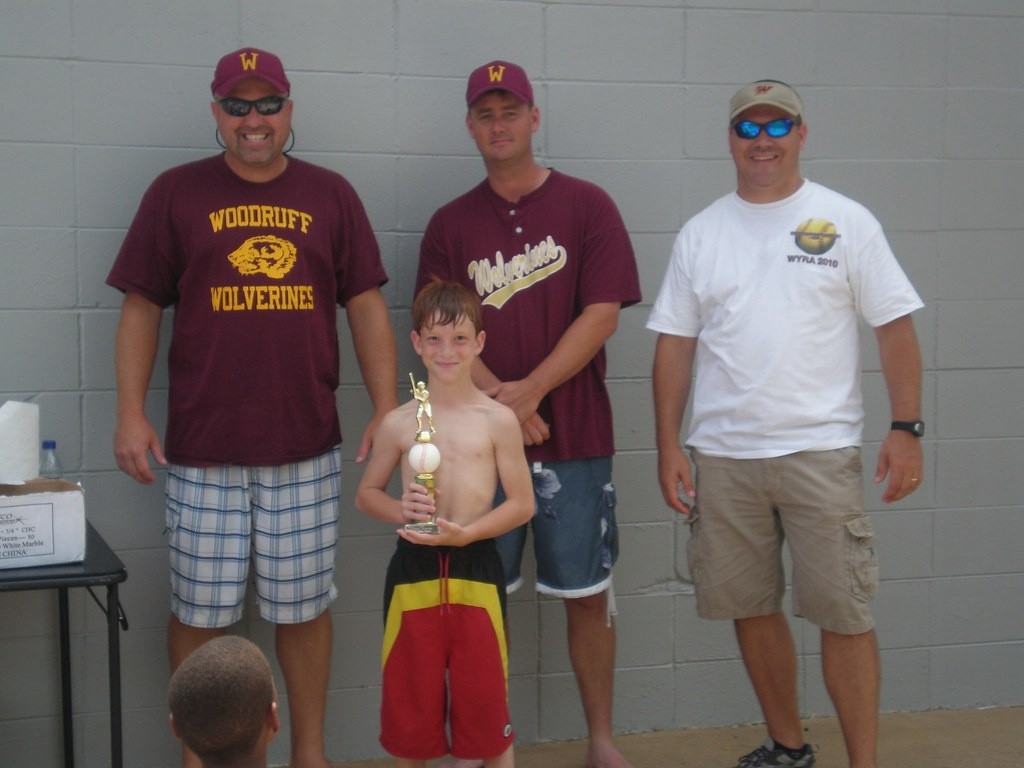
top-left (40, 440), bottom-right (63, 479)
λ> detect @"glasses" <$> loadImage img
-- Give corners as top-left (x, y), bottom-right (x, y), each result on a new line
top-left (732, 118), bottom-right (798, 140)
top-left (216, 95), bottom-right (290, 117)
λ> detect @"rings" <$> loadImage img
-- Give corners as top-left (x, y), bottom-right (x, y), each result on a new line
top-left (911, 478), bottom-right (918, 481)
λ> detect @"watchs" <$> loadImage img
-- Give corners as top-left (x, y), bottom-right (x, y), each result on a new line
top-left (891, 420), bottom-right (925, 437)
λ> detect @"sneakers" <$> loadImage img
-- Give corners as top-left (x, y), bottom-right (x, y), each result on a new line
top-left (735, 735), bottom-right (819, 768)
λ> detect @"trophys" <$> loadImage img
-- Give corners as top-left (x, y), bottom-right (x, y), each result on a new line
top-left (405, 373), bottom-right (440, 534)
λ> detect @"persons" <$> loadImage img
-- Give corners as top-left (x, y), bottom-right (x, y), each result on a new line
top-left (168, 635), bottom-right (281, 768)
top-left (646, 80), bottom-right (926, 768)
top-left (414, 61), bottom-right (643, 768)
top-left (106, 48), bottom-right (399, 768)
top-left (355, 284), bottom-right (536, 768)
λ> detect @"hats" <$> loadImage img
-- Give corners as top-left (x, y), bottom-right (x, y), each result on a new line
top-left (210, 47), bottom-right (291, 97)
top-left (729, 80), bottom-right (805, 126)
top-left (466, 59), bottom-right (533, 108)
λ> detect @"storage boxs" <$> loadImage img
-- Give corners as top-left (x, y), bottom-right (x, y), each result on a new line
top-left (0, 475), bottom-right (85, 571)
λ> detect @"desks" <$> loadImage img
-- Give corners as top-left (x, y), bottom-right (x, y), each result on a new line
top-left (0, 516), bottom-right (129, 768)
